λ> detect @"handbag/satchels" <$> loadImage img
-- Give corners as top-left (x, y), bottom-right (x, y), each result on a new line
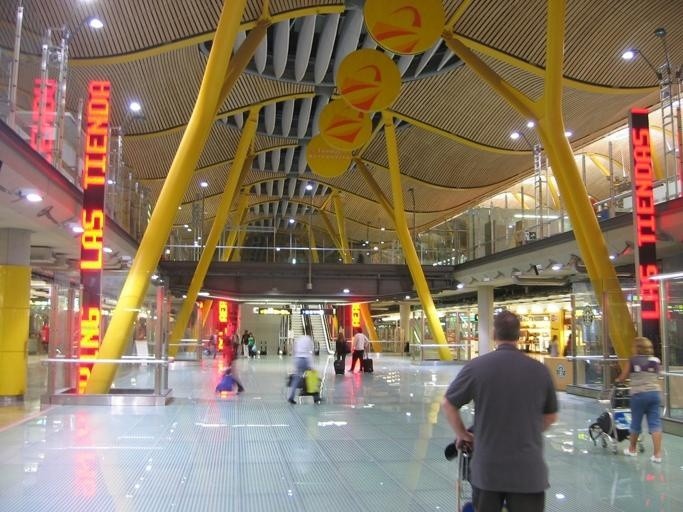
top-left (289, 369), bottom-right (319, 395)
top-left (216, 374), bottom-right (232, 391)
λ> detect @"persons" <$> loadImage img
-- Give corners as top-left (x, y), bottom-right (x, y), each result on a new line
top-left (207, 328), bottom-right (256, 376)
top-left (550, 335), bottom-right (560, 357)
top-left (286, 324), bottom-right (324, 405)
top-left (39, 319), bottom-right (49, 353)
top-left (348, 327), bottom-right (369, 372)
top-left (334, 332), bottom-right (346, 374)
top-left (615, 335), bottom-right (664, 463)
top-left (132, 316), bottom-right (150, 366)
top-left (566, 334), bottom-right (577, 356)
top-left (440, 309), bottom-right (562, 512)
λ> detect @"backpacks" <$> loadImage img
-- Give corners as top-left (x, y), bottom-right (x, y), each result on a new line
top-left (589, 413), bottom-right (629, 441)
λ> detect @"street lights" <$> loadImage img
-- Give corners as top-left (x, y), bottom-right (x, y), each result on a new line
top-left (288, 218), bottom-right (296, 263)
top-left (200, 182), bottom-right (208, 254)
top-left (117, 100), bottom-right (141, 226)
top-left (58, 14), bottom-right (104, 170)
top-left (508, 129), bottom-right (545, 240)
top-left (304, 180), bottom-right (316, 290)
top-left (619, 48), bottom-right (680, 202)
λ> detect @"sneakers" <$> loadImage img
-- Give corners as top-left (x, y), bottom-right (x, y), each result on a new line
top-left (289, 398), bottom-right (296, 403)
top-left (624, 449), bottom-right (637, 456)
top-left (650, 456), bottom-right (661, 462)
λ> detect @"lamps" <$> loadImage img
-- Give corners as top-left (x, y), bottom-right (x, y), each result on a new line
top-left (621, 27), bottom-right (671, 81)
top-left (67, 16), bottom-right (103, 44)
top-left (510, 131), bottom-right (532, 148)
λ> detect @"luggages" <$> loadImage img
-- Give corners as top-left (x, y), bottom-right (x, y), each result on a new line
top-left (363, 359), bottom-right (373, 372)
top-left (334, 360), bottom-right (344, 374)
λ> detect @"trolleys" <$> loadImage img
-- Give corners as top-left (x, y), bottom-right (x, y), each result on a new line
top-left (587, 379), bottom-right (646, 453)
